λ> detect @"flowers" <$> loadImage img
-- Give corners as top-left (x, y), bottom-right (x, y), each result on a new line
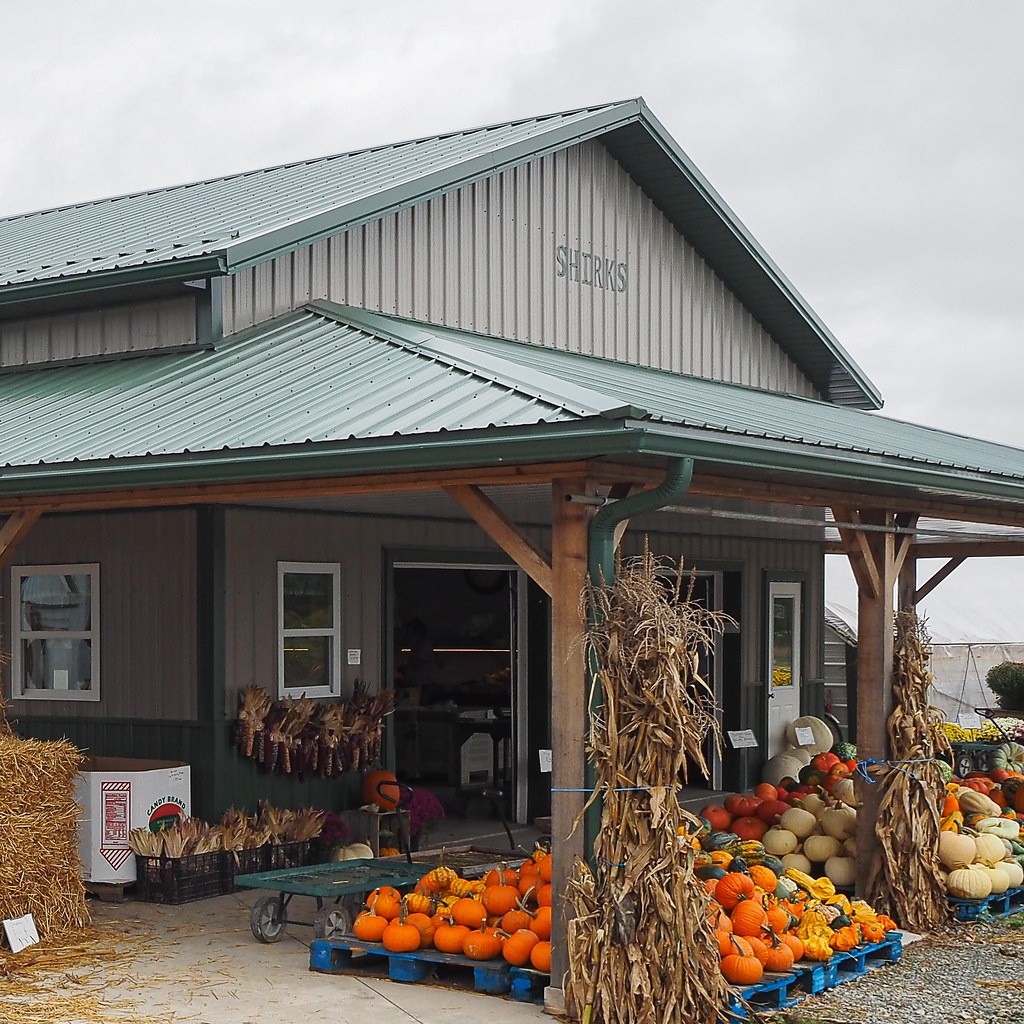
top-left (398, 789), bottom-right (445, 835)
top-left (315, 810), bottom-right (344, 847)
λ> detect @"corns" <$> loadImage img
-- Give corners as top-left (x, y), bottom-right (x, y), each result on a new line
top-left (229, 720), bottom-right (382, 777)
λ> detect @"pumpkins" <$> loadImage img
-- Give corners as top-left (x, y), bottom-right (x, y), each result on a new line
top-left (351, 841), bottom-right (555, 973)
top-left (680, 814), bottom-right (899, 985)
top-left (362, 766), bottom-right (401, 810)
top-left (701, 714), bottom-right (1024, 899)
top-left (329, 843), bottom-right (373, 861)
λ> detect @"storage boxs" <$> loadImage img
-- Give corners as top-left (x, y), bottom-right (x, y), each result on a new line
top-left (264, 836), bottom-right (320, 870)
top-left (135, 851), bottom-right (222, 905)
top-left (71, 755), bottom-right (191, 885)
top-left (221, 845), bottom-right (266, 894)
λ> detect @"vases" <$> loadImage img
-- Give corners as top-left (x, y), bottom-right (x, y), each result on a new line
top-left (312, 838), bottom-right (327, 863)
top-left (408, 828), bottom-right (441, 852)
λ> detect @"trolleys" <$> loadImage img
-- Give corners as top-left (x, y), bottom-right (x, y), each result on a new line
top-left (234, 844), bottom-right (536, 949)
